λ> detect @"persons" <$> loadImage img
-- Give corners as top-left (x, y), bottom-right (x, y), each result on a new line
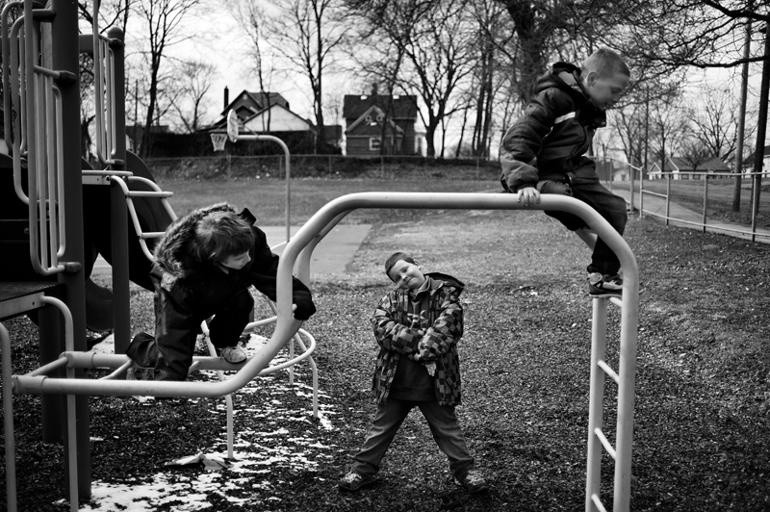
top-left (499, 49), bottom-right (647, 298)
top-left (128, 202), bottom-right (316, 397)
top-left (337, 251), bottom-right (489, 494)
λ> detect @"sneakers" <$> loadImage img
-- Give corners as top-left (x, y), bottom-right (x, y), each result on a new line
top-left (340, 470), bottom-right (368, 489)
top-left (454, 469), bottom-right (489, 493)
top-left (219, 345), bottom-right (248, 364)
top-left (587, 271), bottom-right (645, 297)
